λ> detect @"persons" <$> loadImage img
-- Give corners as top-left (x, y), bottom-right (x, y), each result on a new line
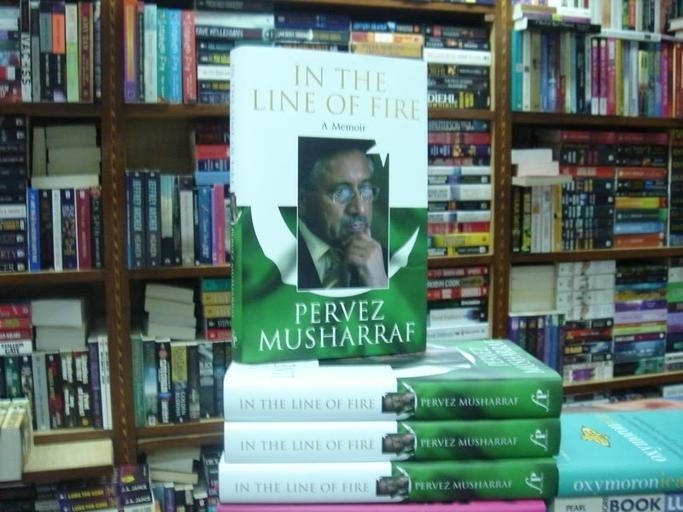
top-left (298, 134), bottom-right (391, 290)
top-left (0, 293), bottom-right (114, 431)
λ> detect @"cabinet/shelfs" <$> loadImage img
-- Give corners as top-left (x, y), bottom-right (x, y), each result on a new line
top-left (325, 0), bottom-right (502, 338)
top-left (501, 0), bottom-right (682, 402)
top-left (1, 0), bottom-right (230, 486)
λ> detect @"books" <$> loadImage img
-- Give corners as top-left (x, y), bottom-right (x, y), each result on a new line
top-left (509, 257), bottom-right (681, 381)
top-left (0, 0), bottom-right (103, 104)
top-left (122, 0), bottom-right (494, 109)
top-left (145, 445), bottom-right (225, 511)
top-left (427, 116), bottom-right (493, 258)
top-left (124, 114), bottom-right (237, 267)
top-left (128, 275), bottom-right (232, 427)
top-left (217, 339), bottom-right (682, 511)
top-left (510, 125), bottom-right (682, 252)
top-left (510, 0), bottom-right (680, 117)
top-left (426, 265), bottom-right (490, 342)
top-left (0, 113), bottom-right (105, 274)
top-left (230, 45), bottom-right (426, 365)
top-left (0, 398), bottom-right (156, 511)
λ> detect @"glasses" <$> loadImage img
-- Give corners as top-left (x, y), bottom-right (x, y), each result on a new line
top-left (306, 187), bottom-right (380, 206)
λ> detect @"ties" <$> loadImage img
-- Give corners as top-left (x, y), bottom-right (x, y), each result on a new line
top-left (323, 251), bottom-right (345, 287)
